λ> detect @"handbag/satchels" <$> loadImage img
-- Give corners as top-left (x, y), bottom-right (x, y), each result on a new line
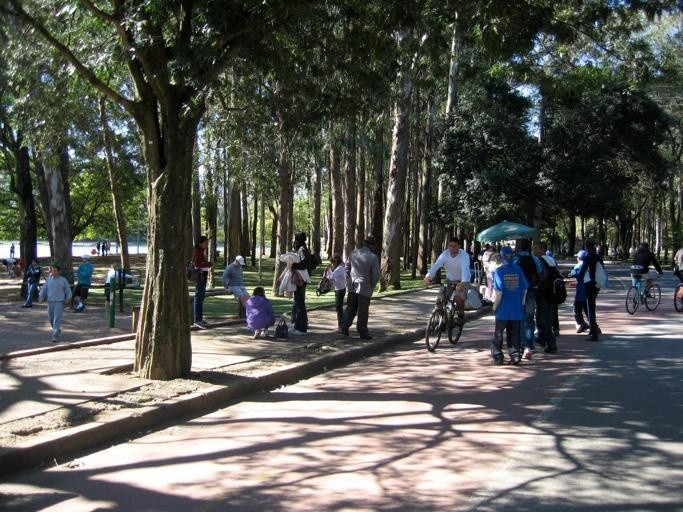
top-left (275, 319), bottom-right (289, 338)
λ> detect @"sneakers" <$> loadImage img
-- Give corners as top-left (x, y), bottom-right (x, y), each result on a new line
top-left (53, 335), bottom-right (57, 341)
top-left (253, 330), bottom-right (259, 338)
top-left (261, 329), bottom-right (268, 336)
top-left (522, 348), bottom-right (531, 358)
top-left (194, 322), bottom-right (207, 329)
top-left (508, 357), bottom-right (520, 365)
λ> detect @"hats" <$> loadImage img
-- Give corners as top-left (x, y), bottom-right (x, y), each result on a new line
top-left (575, 250), bottom-right (587, 258)
top-left (500, 246), bottom-right (513, 266)
top-left (296, 231), bottom-right (307, 240)
top-left (236, 255), bottom-right (245, 265)
top-left (364, 236), bottom-right (377, 247)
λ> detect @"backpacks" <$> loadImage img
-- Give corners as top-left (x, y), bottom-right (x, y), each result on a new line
top-left (309, 253), bottom-right (318, 270)
top-left (539, 256), bottom-right (567, 306)
top-left (595, 262), bottom-right (608, 289)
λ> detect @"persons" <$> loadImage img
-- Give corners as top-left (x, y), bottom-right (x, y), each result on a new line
top-left (513, 238), bottom-right (542, 359)
top-left (423, 237), bottom-right (471, 326)
top-left (631, 242), bottom-right (662, 304)
top-left (554, 304), bottom-right (560, 336)
top-left (290, 232), bottom-right (312, 336)
top-left (96, 240), bottom-right (113, 257)
top-left (10, 243), bottom-right (16, 258)
top-left (0, 263), bottom-right (6, 273)
top-left (188, 236), bottom-right (214, 330)
top-left (222, 256), bottom-right (250, 308)
top-left (567, 250), bottom-right (589, 333)
top-left (516, 254), bottom-right (539, 289)
top-left (597, 242), bottom-right (604, 268)
top-left (22, 261), bottom-right (42, 308)
top-left (39, 266), bottom-right (72, 343)
top-left (340, 237), bottom-right (381, 341)
top-left (11, 262), bottom-right (22, 276)
top-left (492, 247), bottom-right (529, 365)
top-left (246, 287), bottom-right (276, 338)
top-left (673, 248), bottom-right (683, 283)
top-left (534, 242), bottom-right (559, 354)
top-left (74, 255), bottom-right (94, 312)
top-left (323, 254), bottom-right (347, 333)
top-left (579, 239), bottom-right (602, 342)
top-left (104, 263), bottom-right (136, 315)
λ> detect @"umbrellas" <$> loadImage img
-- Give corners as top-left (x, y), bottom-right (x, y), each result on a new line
top-left (476, 220), bottom-right (536, 247)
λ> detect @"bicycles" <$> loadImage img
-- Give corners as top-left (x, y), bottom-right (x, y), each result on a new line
top-left (426, 279), bottom-right (465, 352)
top-left (626, 275), bottom-right (661, 314)
top-left (674, 271), bottom-right (683, 312)
